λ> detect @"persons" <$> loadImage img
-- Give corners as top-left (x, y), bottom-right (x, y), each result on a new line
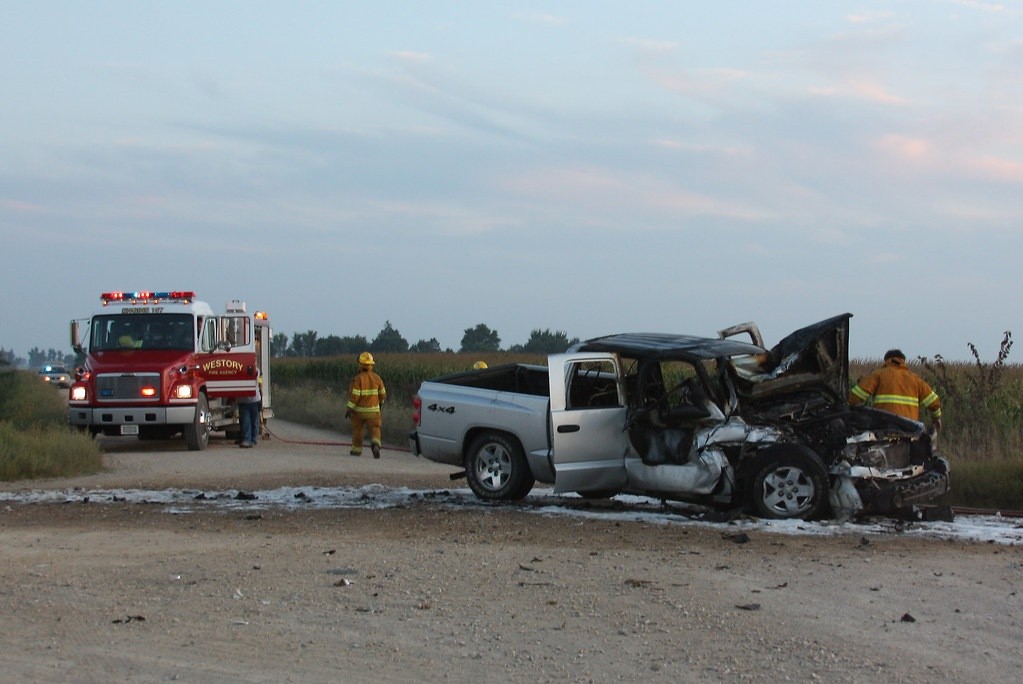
top-left (472, 361), bottom-right (488, 369)
top-left (848, 349), bottom-right (942, 434)
top-left (235, 366), bottom-right (264, 449)
top-left (179, 319), bottom-right (203, 345)
top-left (345, 352), bottom-right (386, 458)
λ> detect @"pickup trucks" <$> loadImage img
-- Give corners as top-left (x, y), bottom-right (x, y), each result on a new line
top-left (409, 310), bottom-right (954, 522)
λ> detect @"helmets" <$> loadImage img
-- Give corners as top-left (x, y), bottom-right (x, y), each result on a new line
top-left (359, 352), bottom-right (375, 365)
top-left (472, 361), bottom-right (488, 369)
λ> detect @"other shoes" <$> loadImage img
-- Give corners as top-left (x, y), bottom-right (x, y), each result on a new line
top-left (373, 446), bottom-right (380, 457)
top-left (240, 441), bottom-right (253, 447)
top-left (350, 451), bottom-right (361, 456)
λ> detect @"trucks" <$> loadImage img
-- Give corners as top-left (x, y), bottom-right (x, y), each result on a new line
top-left (38, 360), bottom-right (71, 387)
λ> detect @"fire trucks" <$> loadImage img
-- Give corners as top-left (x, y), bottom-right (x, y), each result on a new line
top-left (67, 290), bottom-right (274, 452)
